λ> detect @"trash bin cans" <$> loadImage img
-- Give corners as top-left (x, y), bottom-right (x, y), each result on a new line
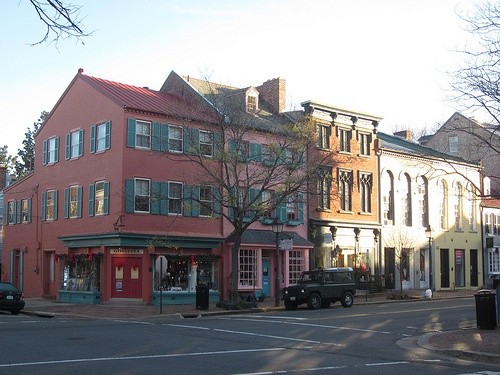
top-left (474, 289), bottom-right (498, 329)
top-left (195, 283), bottom-right (211, 309)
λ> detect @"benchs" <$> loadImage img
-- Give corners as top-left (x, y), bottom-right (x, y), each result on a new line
top-left (354, 290), bottom-right (374, 300)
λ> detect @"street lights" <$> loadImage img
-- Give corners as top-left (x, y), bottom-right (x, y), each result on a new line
top-left (270, 215), bottom-right (284, 308)
top-left (424, 225), bottom-right (435, 297)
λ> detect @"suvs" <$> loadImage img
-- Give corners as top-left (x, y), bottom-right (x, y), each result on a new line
top-left (281, 267), bottom-right (357, 311)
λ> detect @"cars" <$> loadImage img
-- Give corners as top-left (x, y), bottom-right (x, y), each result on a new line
top-left (0, 281), bottom-right (25, 316)
